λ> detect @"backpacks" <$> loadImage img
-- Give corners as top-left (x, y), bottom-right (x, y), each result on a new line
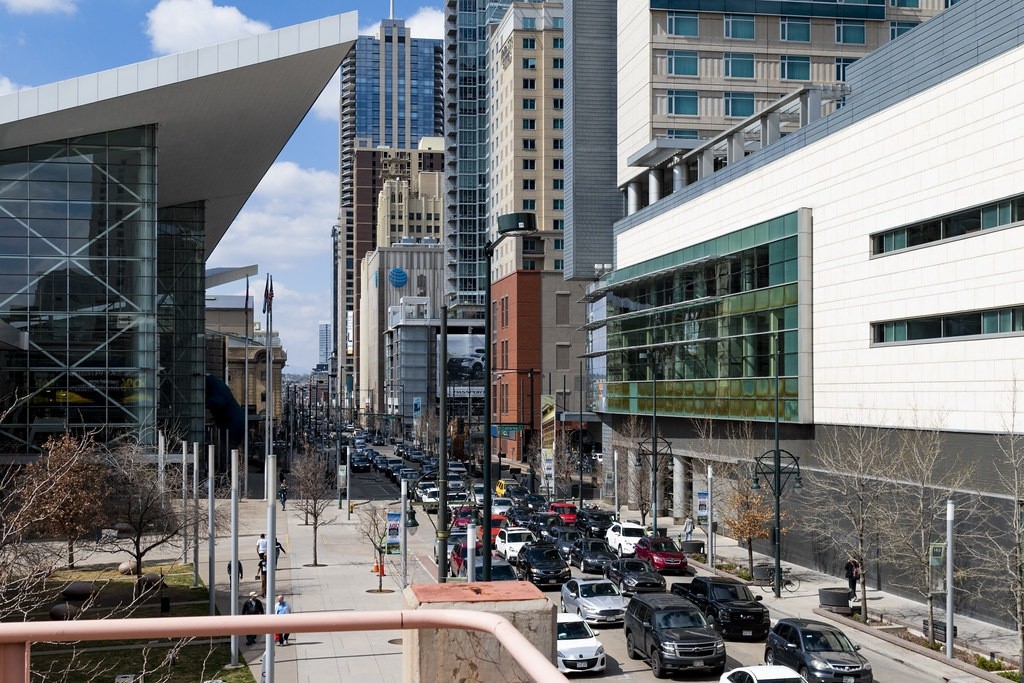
top-left (851, 565), bottom-right (861, 579)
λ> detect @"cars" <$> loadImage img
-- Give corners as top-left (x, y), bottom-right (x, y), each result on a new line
top-left (605, 558), bottom-right (667, 594)
top-left (556, 612), bottom-right (606, 674)
top-left (302, 412), bottom-right (620, 587)
top-left (765, 617), bottom-right (873, 683)
top-left (719, 665), bottom-right (808, 683)
top-left (561, 577), bottom-right (627, 625)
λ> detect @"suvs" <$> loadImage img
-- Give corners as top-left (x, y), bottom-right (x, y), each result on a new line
top-left (636, 536), bottom-right (688, 573)
top-left (606, 521), bottom-right (649, 557)
top-left (623, 593), bottom-right (726, 678)
top-left (448, 352), bottom-right (486, 380)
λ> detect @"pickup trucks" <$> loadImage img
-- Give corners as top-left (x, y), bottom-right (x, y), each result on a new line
top-left (670, 576), bottom-right (770, 641)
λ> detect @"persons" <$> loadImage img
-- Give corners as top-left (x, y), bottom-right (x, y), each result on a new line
top-left (276, 537), bottom-right (285, 569)
top-left (845, 556), bottom-right (860, 602)
top-left (280, 478), bottom-right (288, 489)
top-left (227, 559), bottom-right (244, 590)
top-left (257, 553), bottom-right (267, 597)
top-left (278, 487), bottom-right (287, 511)
top-left (241, 592), bottom-right (264, 645)
top-left (682, 515), bottom-right (695, 541)
top-left (256, 533), bottom-right (266, 559)
top-left (275, 595), bottom-right (293, 646)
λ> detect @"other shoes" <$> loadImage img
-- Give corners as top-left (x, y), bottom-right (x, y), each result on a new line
top-left (246, 639), bottom-right (256, 645)
top-left (275, 640), bottom-right (289, 646)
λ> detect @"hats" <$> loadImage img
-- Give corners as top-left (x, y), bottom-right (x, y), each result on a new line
top-left (249, 591), bottom-right (259, 597)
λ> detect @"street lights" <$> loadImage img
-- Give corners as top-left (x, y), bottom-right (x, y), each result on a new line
top-left (314, 380), bottom-right (324, 437)
top-left (634, 436), bottom-right (675, 537)
top-left (327, 374), bottom-right (337, 447)
top-left (286, 385), bottom-right (308, 436)
top-left (752, 449), bottom-right (803, 598)
top-left (401, 479), bottom-right (419, 593)
top-left (307, 385), bottom-right (316, 435)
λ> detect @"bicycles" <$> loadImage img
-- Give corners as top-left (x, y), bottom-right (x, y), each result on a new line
top-left (760, 567), bottom-right (800, 592)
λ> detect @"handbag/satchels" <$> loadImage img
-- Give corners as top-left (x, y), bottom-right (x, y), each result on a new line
top-left (274, 633), bottom-right (280, 642)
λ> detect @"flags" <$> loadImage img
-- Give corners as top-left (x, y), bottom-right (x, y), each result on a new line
top-left (386, 512), bottom-right (401, 555)
top-left (338, 465), bottom-right (347, 487)
top-left (697, 492), bottom-right (709, 525)
top-left (929, 543), bottom-right (946, 593)
